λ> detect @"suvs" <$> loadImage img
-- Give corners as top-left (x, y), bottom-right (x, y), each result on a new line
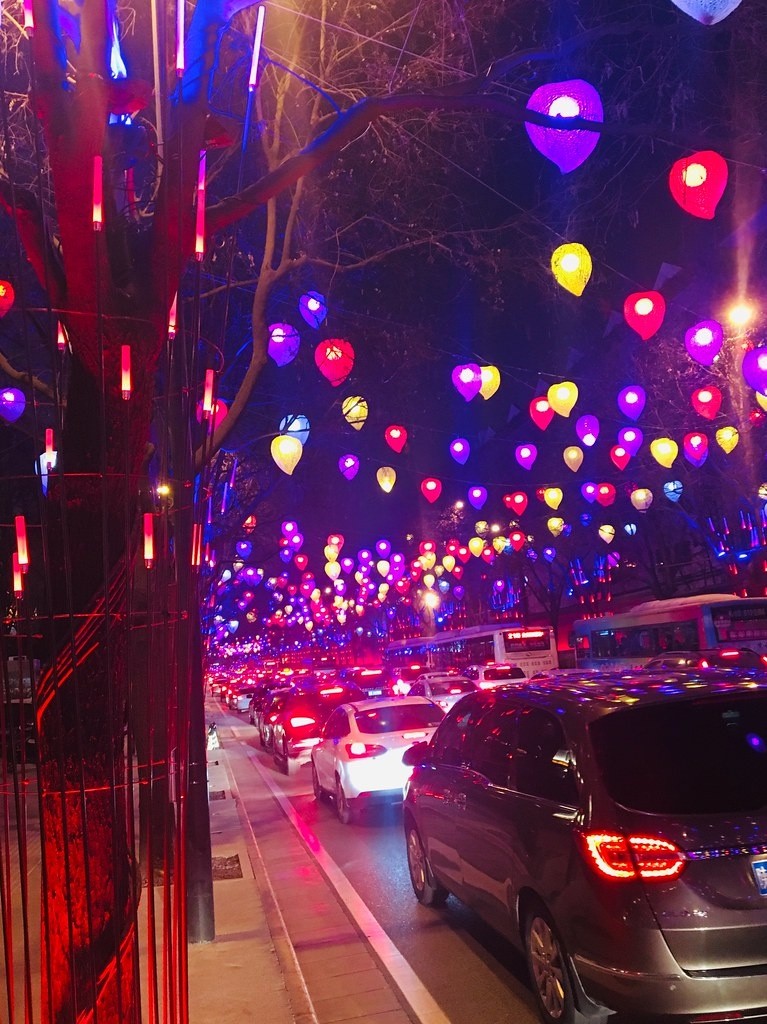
top-left (400, 675), bottom-right (766, 1022)
top-left (645, 647), bottom-right (767, 678)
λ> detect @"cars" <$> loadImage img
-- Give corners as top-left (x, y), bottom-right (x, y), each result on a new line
top-left (311, 694), bottom-right (457, 824)
top-left (201, 660), bottom-right (537, 774)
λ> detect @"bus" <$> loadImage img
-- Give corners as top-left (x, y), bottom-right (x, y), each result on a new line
top-left (377, 622), bottom-right (558, 679)
top-left (568, 594), bottom-right (767, 682)
